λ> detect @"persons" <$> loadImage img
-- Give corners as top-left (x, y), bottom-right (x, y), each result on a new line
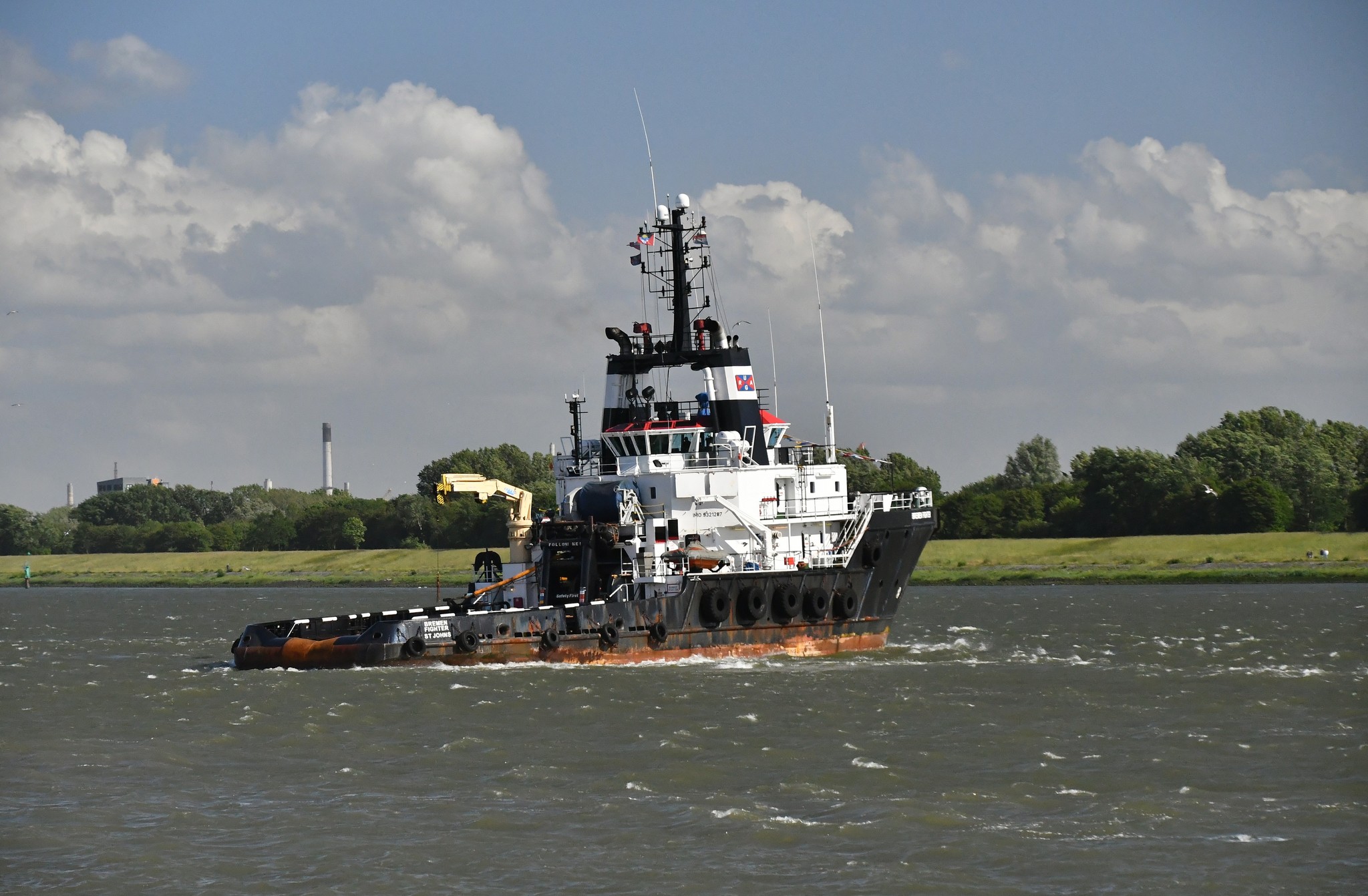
top-left (1320, 549), bottom-right (1328, 559)
top-left (1307, 550), bottom-right (1313, 558)
top-left (634, 342), bottom-right (642, 355)
top-left (683, 436), bottom-right (691, 453)
top-left (227, 564), bottom-right (229, 569)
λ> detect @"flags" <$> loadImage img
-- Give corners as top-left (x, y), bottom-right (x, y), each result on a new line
top-left (637, 233), bottom-right (653, 246)
top-left (693, 234), bottom-right (709, 245)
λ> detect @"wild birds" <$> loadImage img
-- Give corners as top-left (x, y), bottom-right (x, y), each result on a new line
top-left (731, 320), bottom-right (752, 333)
top-left (242, 566), bottom-right (252, 572)
top-left (1201, 483), bottom-right (1219, 497)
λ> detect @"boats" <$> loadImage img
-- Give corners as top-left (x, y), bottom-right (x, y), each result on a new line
top-left (230, 87), bottom-right (937, 676)
